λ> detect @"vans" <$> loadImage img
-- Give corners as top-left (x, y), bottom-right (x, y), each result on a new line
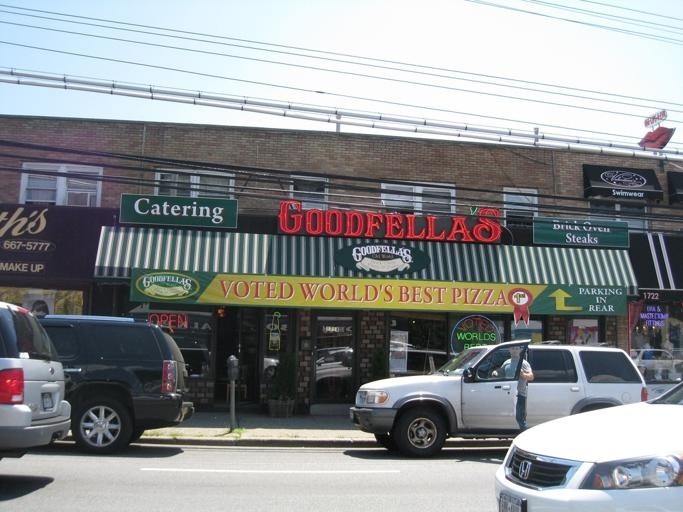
top-left (0, 302), bottom-right (195, 455)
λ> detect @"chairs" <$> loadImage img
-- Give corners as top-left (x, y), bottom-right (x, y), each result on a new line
top-left (226, 365), bottom-right (248, 403)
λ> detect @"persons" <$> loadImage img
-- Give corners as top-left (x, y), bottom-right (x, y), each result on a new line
top-left (490, 345), bottom-right (534, 430)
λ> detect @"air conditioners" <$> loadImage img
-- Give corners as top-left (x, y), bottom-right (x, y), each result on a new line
top-left (66, 191), bottom-right (91, 208)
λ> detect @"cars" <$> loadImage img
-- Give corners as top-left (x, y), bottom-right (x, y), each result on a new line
top-left (494, 382), bottom-right (683, 512)
top-left (350, 339), bottom-right (647, 458)
top-left (631, 349), bottom-right (683, 379)
top-left (260, 342), bottom-right (459, 403)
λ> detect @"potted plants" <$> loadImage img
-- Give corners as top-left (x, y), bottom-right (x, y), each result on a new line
top-left (265, 349), bottom-right (298, 418)
